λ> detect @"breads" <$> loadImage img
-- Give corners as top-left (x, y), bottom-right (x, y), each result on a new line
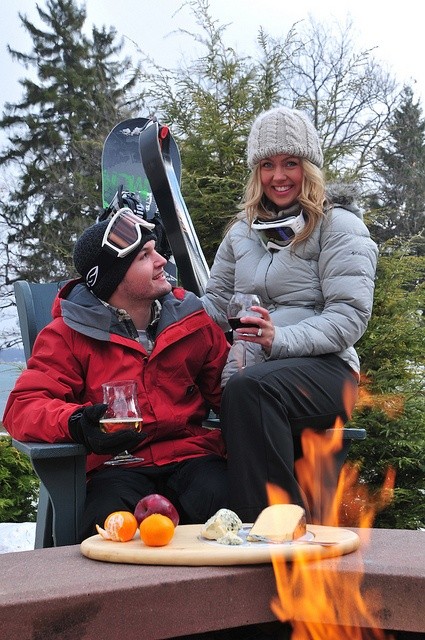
top-left (247, 501), bottom-right (308, 542)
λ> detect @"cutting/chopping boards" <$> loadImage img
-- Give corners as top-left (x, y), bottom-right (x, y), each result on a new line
top-left (79, 523), bottom-right (356, 570)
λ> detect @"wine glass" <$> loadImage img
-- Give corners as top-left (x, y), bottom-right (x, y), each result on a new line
top-left (228, 292), bottom-right (262, 369)
top-left (98, 380), bottom-right (144, 467)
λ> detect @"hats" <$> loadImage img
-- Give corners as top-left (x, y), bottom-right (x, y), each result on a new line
top-left (246, 106), bottom-right (324, 170)
top-left (324, 139), bottom-right (326, 139)
top-left (73, 218), bottom-right (158, 303)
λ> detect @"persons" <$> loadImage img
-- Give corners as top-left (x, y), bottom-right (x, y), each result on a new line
top-left (3, 208), bottom-right (232, 540)
top-left (198, 107), bottom-right (381, 515)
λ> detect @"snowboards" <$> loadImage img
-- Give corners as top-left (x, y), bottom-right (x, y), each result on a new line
top-left (97, 115), bottom-right (185, 292)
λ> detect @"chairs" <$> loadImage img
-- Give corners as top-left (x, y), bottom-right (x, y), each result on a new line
top-left (14, 272), bottom-right (368, 549)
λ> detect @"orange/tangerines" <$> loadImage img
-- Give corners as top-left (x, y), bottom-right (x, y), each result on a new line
top-left (138, 511), bottom-right (176, 548)
top-left (94, 510), bottom-right (138, 543)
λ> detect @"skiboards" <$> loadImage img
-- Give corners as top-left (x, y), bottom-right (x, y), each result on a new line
top-left (138, 119), bottom-right (233, 345)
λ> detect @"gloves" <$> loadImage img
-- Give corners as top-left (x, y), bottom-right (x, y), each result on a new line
top-left (68, 403), bottom-right (150, 455)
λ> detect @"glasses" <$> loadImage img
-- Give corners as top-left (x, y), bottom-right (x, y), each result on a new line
top-left (83, 207), bottom-right (156, 290)
top-left (250, 209), bottom-right (306, 252)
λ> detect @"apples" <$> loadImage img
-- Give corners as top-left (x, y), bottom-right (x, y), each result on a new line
top-left (133, 492), bottom-right (180, 530)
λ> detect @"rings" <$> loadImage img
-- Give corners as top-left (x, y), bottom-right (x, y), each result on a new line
top-left (254, 325), bottom-right (264, 339)
